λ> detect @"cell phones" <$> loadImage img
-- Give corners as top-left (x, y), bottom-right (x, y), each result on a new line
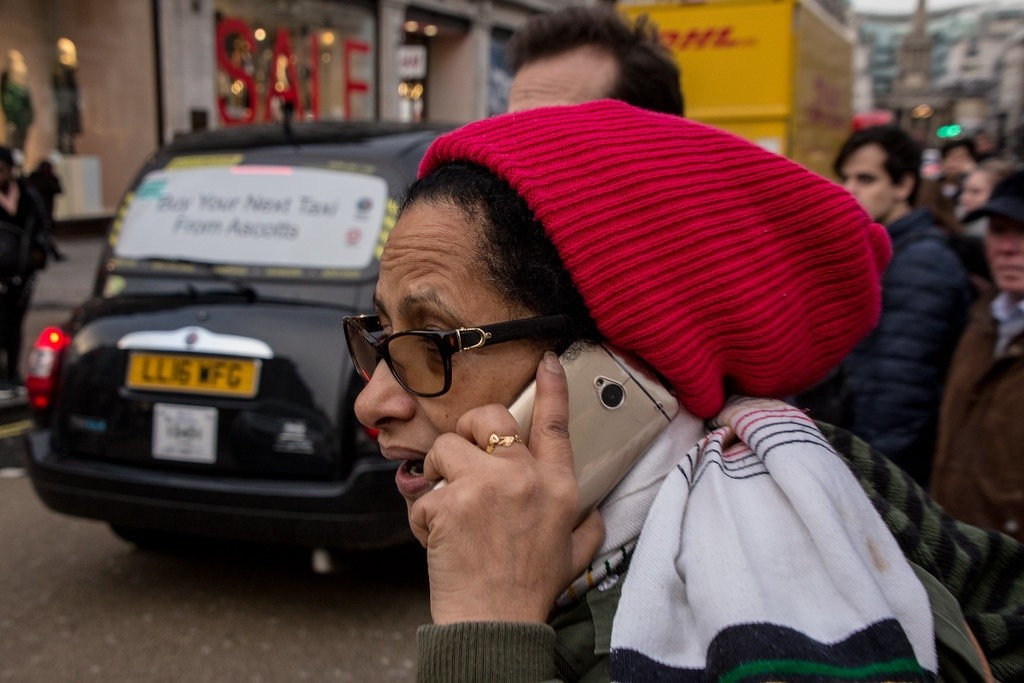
top-left (429, 337), bottom-right (682, 531)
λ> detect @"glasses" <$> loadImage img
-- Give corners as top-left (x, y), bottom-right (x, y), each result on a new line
top-left (340, 315), bottom-right (594, 398)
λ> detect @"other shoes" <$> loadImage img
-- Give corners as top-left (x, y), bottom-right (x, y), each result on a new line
top-left (0, 373), bottom-right (26, 399)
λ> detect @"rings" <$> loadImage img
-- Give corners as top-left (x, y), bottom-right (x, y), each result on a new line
top-left (486, 434), bottom-right (524, 454)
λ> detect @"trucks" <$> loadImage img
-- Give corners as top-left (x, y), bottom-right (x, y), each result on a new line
top-left (611, 0), bottom-right (858, 184)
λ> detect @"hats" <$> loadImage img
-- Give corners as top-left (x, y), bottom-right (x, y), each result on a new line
top-left (416, 99), bottom-right (893, 420)
top-left (943, 135), bottom-right (978, 158)
top-left (853, 110), bottom-right (892, 129)
top-left (959, 169), bottom-right (1024, 226)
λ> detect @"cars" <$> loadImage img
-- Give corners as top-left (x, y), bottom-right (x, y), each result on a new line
top-left (18, 116), bottom-right (470, 584)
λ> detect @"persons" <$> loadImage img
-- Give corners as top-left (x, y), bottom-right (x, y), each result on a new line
top-left (0, 147), bottom-right (51, 400)
top-left (0, 50), bottom-right (36, 166)
top-left (49, 38), bottom-right (85, 156)
top-left (353, 100), bottom-right (1024, 682)
top-left (816, 125), bottom-right (1024, 542)
top-left (503, 7), bottom-right (684, 117)
